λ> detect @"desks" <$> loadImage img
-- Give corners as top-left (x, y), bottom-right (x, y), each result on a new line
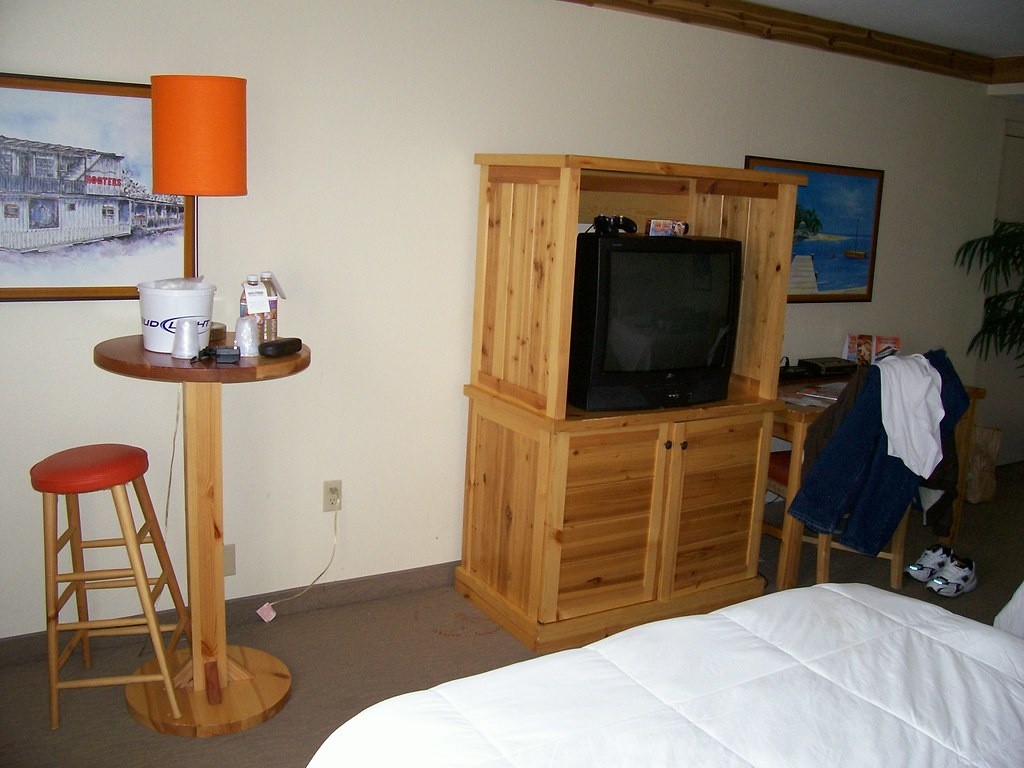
top-left (94, 328), bottom-right (311, 737)
top-left (761, 372), bottom-right (987, 591)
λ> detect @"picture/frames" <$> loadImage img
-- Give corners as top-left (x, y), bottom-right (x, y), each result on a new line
top-left (0, 70), bottom-right (198, 302)
top-left (744, 154), bottom-right (883, 303)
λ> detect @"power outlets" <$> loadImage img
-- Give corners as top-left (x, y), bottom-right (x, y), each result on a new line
top-left (322, 480), bottom-right (343, 512)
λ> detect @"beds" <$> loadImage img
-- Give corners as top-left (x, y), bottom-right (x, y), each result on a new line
top-left (297, 584), bottom-right (1024, 768)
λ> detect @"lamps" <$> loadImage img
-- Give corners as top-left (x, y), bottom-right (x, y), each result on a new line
top-left (150, 76), bottom-right (247, 339)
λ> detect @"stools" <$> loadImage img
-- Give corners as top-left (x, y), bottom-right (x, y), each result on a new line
top-left (30, 444), bottom-right (187, 731)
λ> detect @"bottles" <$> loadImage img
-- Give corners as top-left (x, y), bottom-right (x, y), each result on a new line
top-left (259, 273), bottom-right (278, 342)
top-left (240, 275), bottom-right (264, 344)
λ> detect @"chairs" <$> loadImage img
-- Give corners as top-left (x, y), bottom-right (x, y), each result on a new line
top-left (803, 349), bottom-right (970, 595)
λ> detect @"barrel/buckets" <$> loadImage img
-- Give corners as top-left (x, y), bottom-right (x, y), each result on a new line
top-left (136, 278), bottom-right (216, 354)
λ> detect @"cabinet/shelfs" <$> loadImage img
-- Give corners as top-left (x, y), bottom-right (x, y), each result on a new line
top-left (455, 150), bottom-right (809, 649)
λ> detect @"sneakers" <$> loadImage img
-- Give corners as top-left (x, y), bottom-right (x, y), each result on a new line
top-left (925, 556), bottom-right (977, 597)
top-left (903, 543), bottom-right (954, 582)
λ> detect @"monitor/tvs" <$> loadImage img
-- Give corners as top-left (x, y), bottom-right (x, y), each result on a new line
top-left (565, 232), bottom-right (742, 416)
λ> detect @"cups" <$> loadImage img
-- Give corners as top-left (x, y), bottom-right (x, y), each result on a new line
top-left (171, 319), bottom-right (200, 360)
top-left (233, 317), bottom-right (260, 357)
